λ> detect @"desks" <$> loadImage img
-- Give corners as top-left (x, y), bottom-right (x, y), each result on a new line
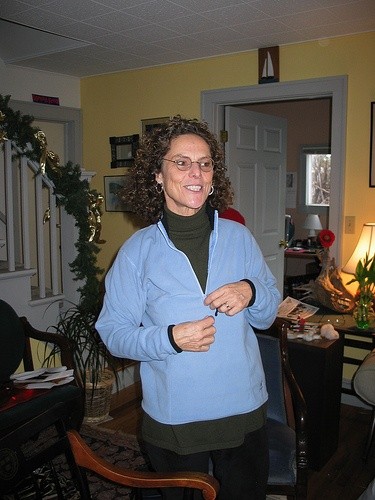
top-left (304, 299), bottom-right (375, 396)
top-left (286, 327), bottom-right (345, 472)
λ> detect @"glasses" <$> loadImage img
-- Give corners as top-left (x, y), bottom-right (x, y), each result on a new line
top-left (320, 308), bottom-right (345, 324)
top-left (161, 157), bottom-right (214, 172)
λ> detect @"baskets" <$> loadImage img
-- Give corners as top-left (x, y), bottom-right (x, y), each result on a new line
top-left (83, 370), bottom-right (114, 422)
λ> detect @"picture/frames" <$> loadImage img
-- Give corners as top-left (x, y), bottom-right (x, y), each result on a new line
top-left (369, 102), bottom-right (375, 188)
top-left (140, 116), bottom-right (170, 139)
top-left (109, 134), bottom-right (140, 169)
top-left (103, 175), bottom-right (137, 214)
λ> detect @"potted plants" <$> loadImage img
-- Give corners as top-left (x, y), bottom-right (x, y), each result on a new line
top-left (39, 296), bottom-right (128, 427)
top-left (346, 254), bottom-right (375, 330)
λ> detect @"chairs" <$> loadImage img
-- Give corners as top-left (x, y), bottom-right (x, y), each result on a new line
top-left (0, 298), bottom-right (220, 500)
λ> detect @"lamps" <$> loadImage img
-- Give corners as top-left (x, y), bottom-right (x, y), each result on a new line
top-left (301, 213), bottom-right (323, 238)
top-left (341, 222), bottom-right (375, 276)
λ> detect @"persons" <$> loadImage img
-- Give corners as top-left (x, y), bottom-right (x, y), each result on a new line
top-left (94, 114), bottom-right (281, 500)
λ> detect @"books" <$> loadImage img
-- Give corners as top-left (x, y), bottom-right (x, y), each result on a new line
top-left (277, 296), bottom-right (319, 322)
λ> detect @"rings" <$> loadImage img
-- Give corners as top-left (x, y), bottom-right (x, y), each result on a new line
top-left (225, 305), bottom-right (230, 310)
